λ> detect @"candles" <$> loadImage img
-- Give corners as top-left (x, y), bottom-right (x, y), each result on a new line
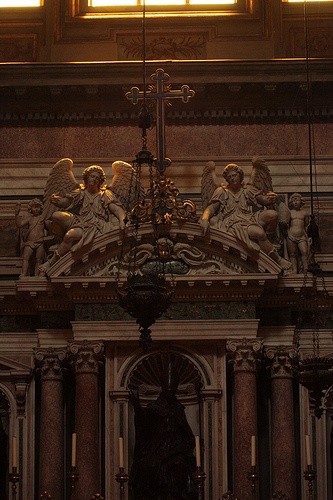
top-left (306, 435), bottom-right (311, 465)
top-left (251, 436), bottom-right (255, 466)
top-left (71, 433), bottom-right (76, 466)
top-left (195, 436), bottom-right (201, 467)
top-left (119, 437), bottom-right (123, 467)
top-left (12, 436), bottom-right (17, 467)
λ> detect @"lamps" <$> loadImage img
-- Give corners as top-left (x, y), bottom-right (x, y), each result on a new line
top-left (265, 3), bottom-right (333, 420)
top-left (114, 0), bottom-right (175, 337)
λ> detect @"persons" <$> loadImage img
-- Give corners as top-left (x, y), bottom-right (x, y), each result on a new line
top-left (154, 236), bottom-right (174, 262)
top-left (13, 198), bottom-right (49, 276)
top-left (196, 161), bottom-right (292, 270)
top-left (280, 193), bottom-right (314, 274)
top-left (40, 158), bottom-right (143, 273)
